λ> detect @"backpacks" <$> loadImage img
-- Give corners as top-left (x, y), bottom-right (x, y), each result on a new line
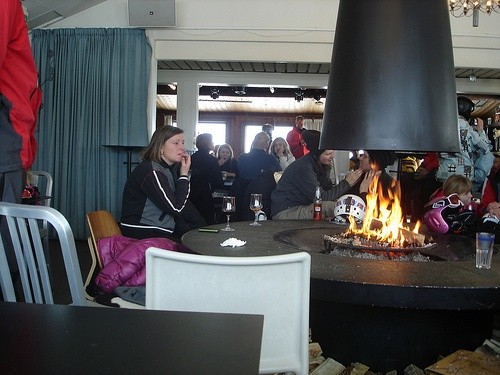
top-left (435, 148), bottom-right (474, 182)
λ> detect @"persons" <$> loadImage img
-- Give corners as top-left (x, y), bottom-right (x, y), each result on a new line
top-left (0, 0), bottom-right (43, 302)
top-left (190, 97), bottom-right (500, 245)
top-left (119, 125), bottom-right (191, 243)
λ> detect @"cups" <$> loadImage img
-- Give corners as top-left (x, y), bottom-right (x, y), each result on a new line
top-left (475, 232), bottom-right (495, 270)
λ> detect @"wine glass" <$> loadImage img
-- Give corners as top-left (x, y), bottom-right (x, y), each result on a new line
top-left (222, 196), bottom-right (236, 232)
top-left (249, 194), bottom-right (263, 226)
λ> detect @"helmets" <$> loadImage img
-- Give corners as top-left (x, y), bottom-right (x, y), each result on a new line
top-left (425, 194), bottom-right (464, 233)
top-left (334, 194), bottom-right (366, 225)
top-left (457, 96), bottom-right (475, 121)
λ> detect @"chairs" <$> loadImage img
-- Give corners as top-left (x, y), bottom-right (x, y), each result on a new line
top-left (144, 247), bottom-right (311, 375)
top-left (2, 170), bottom-right (54, 297)
top-left (82, 210), bottom-right (146, 310)
top-left (0, 202), bottom-right (112, 308)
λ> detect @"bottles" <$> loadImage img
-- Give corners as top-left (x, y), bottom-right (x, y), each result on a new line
top-left (313, 187), bottom-right (322, 220)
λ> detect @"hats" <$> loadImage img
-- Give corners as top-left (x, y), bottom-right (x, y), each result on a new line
top-left (302, 130), bottom-right (326, 160)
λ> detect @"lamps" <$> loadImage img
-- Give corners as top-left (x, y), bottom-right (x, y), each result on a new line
top-left (295, 90), bottom-right (304, 101)
top-left (314, 92), bottom-right (323, 104)
top-left (447, 0), bottom-right (500, 27)
top-left (211, 87), bottom-right (220, 99)
top-left (234, 87), bottom-right (248, 95)
